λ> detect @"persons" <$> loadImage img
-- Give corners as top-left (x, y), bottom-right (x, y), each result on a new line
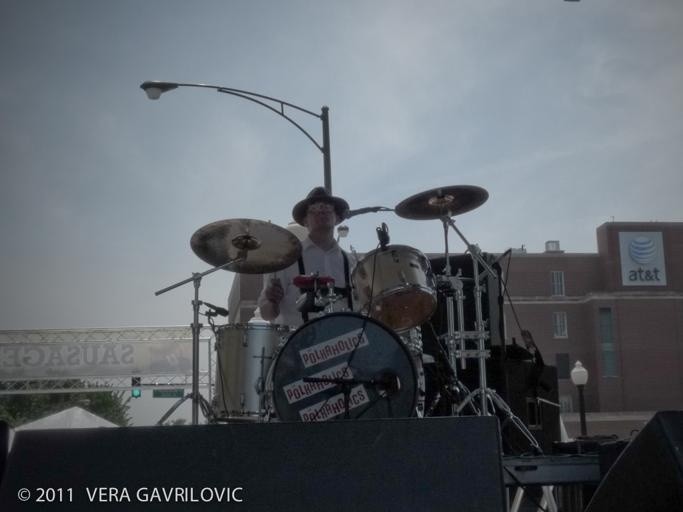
top-left (258, 187), bottom-right (361, 330)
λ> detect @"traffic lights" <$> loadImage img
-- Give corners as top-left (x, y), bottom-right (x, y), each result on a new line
top-left (132, 377), bottom-right (140, 396)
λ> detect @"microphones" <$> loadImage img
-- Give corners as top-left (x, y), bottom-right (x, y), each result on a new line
top-left (374, 375), bottom-right (402, 392)
top-left (204, 301), bottom-right (229, 316)
top-left (343, 206), bottom-right (381, 218)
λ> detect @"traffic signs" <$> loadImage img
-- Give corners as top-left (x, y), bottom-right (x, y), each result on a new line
top-left (152, 389), bottom-right (183, 398)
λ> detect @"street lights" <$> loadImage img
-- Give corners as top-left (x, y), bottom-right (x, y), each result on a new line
top-left (140, 79), bottom-right (331, 198)
top-left (570, 361), bottom-right (590, 438)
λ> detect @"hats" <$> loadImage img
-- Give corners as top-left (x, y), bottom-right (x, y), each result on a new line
top-left (292, 187), bottom-right (350, 227)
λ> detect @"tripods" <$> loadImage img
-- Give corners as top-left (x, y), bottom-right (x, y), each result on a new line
top-left (156, 324), bottom-right (216, 426)
top-left (454, 249), bottom-right (544, 455)
top-left (424, 275), bottom-right (481, 417)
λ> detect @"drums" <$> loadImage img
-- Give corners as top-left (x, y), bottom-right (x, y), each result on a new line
top-left (352, 244), bottom-right (437, 333)
top-left (217, 324), bottom-right (290, 421)
top-left (265, 311), bottom-right (425, 422)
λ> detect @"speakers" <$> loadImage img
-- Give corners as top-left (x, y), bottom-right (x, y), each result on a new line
top-left (0, 416), bottom-right (507, 511)
top-left (583, 411), bottom-right (681, 511)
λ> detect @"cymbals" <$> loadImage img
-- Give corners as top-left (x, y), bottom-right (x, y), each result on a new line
top-left (396, 185), bottom-right (488, 220)
top-left (189, 218), bottom-right (302, 275)
